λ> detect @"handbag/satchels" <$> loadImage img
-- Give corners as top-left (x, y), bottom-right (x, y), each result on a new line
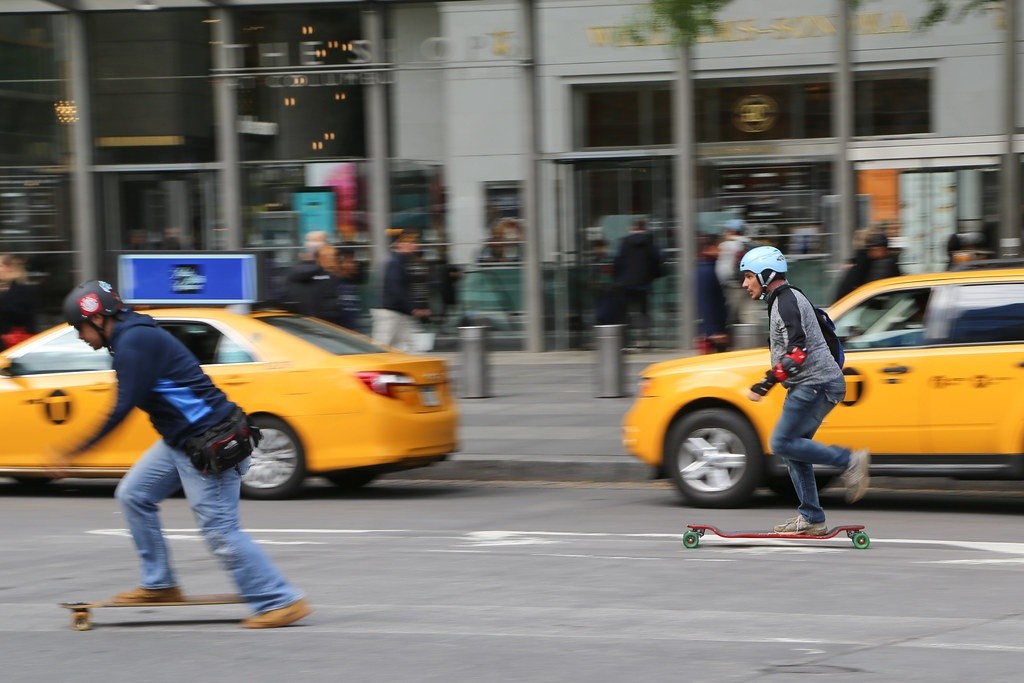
top-left (181, 407), bottom-right (262, 474)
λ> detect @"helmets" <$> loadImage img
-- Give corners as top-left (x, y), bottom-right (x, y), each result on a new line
top-left (61, 280), bottom-right (122, 324)
top-left (739, 245), bottom-right (788, 274)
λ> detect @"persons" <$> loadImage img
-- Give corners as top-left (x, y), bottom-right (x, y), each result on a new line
top-left (55, 279), bottom-right (313, 629)
top-left (273, 228), bottom-right (431, 352)
top-left (696, 218), bottom-right (775, 334)
top-left (0, 252), bottom-right (51, 351)
top-left (612, 218), bottom-right (664, 343)
top-left (740, 246), bottom-right (869, 536)
top-left (838, 234), bottom-right (902, 301)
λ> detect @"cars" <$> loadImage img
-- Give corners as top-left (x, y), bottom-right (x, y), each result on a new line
top-left (0, 253), bottom-right (462, 500)
top-left (621, 259), bottom-right (1024, 508)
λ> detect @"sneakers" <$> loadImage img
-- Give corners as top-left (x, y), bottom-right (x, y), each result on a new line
top-left (773, 515), bottom-right (828, 536)
top-left (840, 450), bottom-right (869, 504)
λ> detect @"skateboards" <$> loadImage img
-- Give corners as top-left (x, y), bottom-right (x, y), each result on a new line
top-left (682, 524), bottom-right (870, 550)
top-left (56, 593), bottom-right (248, 631)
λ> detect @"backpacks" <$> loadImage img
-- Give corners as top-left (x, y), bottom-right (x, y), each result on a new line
top-left (767, 285), bottom-right (845, 369)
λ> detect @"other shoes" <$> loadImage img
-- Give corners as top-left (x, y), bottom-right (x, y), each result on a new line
top-left (241, 598), bottom-right (312, 629)
top-left (112, 588), bottom-right (182, 604)
